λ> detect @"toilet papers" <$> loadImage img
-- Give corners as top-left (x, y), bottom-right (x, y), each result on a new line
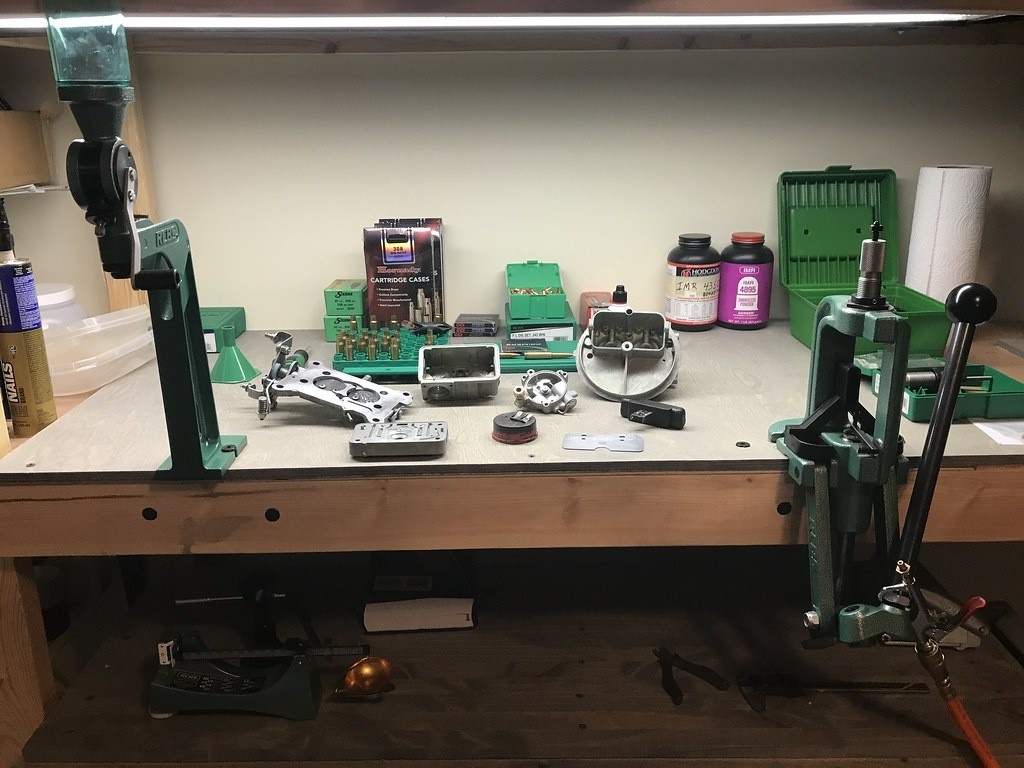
top-left (904, 164), bottom-right (994, 304)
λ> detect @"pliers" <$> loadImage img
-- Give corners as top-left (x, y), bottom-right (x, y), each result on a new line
top-left (653, 645), bottom-right (730, 706)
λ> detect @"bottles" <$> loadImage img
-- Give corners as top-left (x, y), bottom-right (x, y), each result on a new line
top-left (612, 285), bottom-right (627, 311)
top-left (666, 233), bottom-right (720, 331)
top-left (720, 232), bottom-right (774, 331)
top-left (0, 201), bottom-right (57, 438)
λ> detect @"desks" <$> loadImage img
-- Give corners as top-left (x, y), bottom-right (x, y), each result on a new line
top-left (0, 322), bottom-right (1024, 768)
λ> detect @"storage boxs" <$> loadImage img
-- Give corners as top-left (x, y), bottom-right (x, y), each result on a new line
top-left (199, 307), bottom-right (246, 352)
top-left (45, 304), bottom-right (158, 397)
top-left (776, 165), bottom-right (953, 360)
top-left (504, 260), bottom-right (577, 342)
top-left (855, 358), bottom-right (1024, 422)
top-left (324, 279), bottom-right (369, 316)
top-left (324, 315), bottom-right (368, 342)
